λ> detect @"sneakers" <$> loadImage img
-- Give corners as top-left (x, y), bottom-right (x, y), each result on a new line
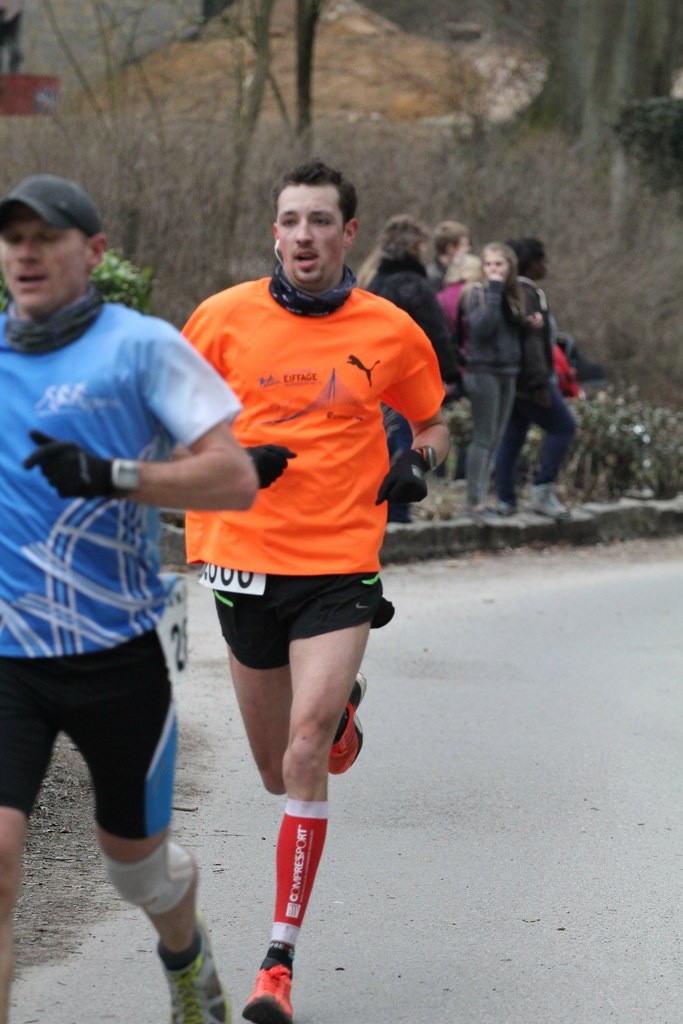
top-left (158, 910), bottom-right (230, 1024)
top-left (242, 958), bottom-right (293, 1024)
top-left (328, 671), bottom-right (367, 775)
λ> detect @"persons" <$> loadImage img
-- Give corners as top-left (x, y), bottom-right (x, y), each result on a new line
top-left (357, 217), bottom-right (577, 523)
top-left (159, 160), bottom-right (455, 1023)
top-left (0, 174), bottom-right (230, 1024)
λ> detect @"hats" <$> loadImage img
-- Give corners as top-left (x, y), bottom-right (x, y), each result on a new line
top-left (0, 174), bottom-right (102, 236)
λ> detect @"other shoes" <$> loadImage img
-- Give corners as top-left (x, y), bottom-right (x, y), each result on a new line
top-left (498, 500), bottom-right (518, 515)
top-left (533, 491), bottom-right (572, 521)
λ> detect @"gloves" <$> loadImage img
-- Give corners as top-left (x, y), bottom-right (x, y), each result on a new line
top-left (244, 444), bottom-right (297, 489)
top-left (376, 449), bottom-right (430, 506)
top-left (22, 429), bottom-right (114, 500)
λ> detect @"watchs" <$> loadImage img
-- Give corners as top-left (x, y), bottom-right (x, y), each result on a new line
top-left (108, 459), bottom-right (144, 500)
top-left (413, 444), bottom-right (438, 471)
